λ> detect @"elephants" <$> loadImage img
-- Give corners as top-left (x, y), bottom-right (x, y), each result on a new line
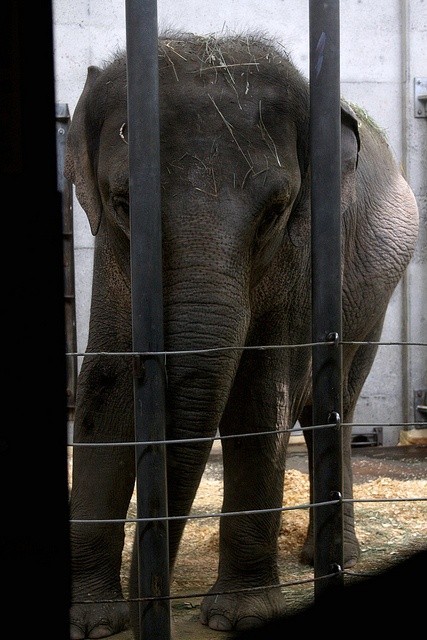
top-left (64, 28), bottom-right (420, 639)
